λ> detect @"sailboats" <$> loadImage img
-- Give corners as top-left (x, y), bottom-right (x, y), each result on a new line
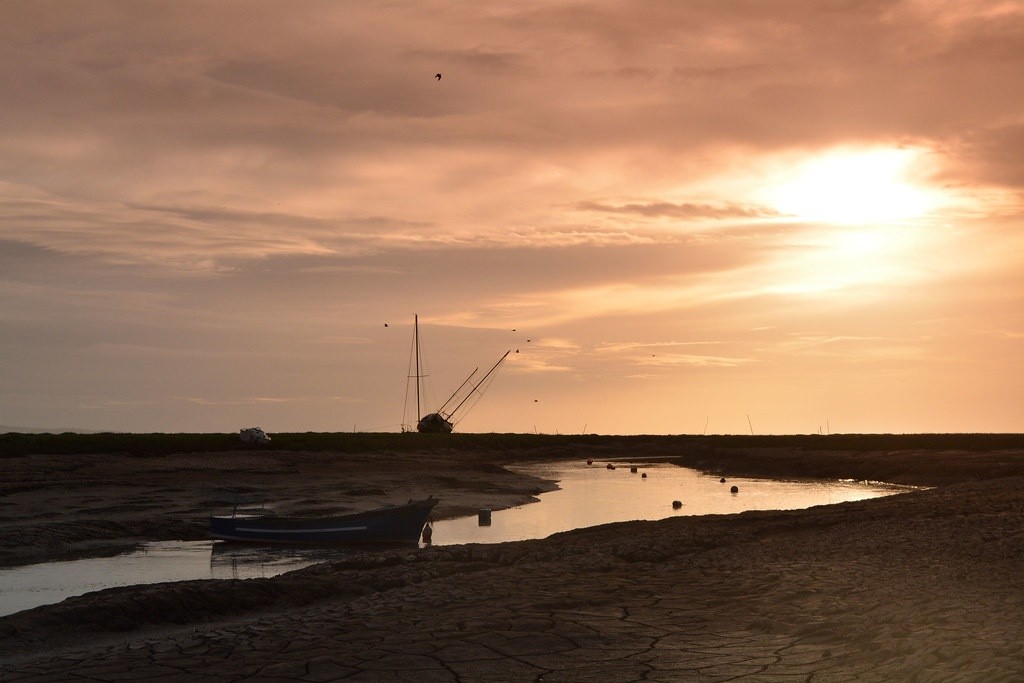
top-left (400, 312), bottom-right (511, 434)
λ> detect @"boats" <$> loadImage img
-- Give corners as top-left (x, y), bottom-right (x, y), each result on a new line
top-left (205, 493), bottom-right (441, 547)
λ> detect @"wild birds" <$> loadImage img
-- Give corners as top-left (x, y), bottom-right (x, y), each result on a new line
top-left (385, 324), bottom-right (656, 402)
top-left (435, 73), bottom-right (443, 81)
